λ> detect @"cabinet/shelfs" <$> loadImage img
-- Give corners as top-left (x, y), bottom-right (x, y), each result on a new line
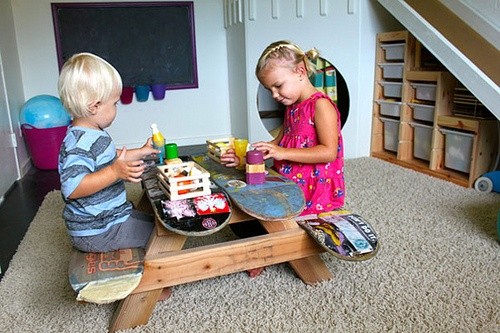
top-left (369, 30), bottom-right (500, 189)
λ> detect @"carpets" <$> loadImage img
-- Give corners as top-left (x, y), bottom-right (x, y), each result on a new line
top-left (1, 157), bottom-right (499, 333)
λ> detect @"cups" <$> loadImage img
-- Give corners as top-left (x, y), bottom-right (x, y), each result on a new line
top-left (120, 86), bottom-right (134, 104)
top-left (233, 139), bottom-right (248, 170)
top-left (135, 84), bottom-right (150, 102)
top-left (151, 83), bottom-right (166, 100)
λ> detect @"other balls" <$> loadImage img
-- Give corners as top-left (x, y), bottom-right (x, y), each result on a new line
top-left (19, 95), bottom-right (72, 129)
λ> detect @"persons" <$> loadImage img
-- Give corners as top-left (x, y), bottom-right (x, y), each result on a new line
top-left (57, 52), bottom-right (172, 301)
top-left (221, 40), bottom-right (345, 278)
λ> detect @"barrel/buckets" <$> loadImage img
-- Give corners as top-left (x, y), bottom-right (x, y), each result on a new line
top-left (20, 120), bottom-right (74, 170)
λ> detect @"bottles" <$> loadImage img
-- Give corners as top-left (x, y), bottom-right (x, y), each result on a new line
top-left (150, 123), bottom-right (166, 165)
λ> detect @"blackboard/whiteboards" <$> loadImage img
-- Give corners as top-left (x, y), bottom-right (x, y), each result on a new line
top-left (51, 1), bottom-right (199, 92)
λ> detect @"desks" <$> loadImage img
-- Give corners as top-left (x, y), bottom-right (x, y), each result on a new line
top-left (108, 155), bottom-right (333, 333)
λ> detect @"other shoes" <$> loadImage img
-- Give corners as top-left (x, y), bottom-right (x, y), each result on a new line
top-left (246, 265), bottom-right (264, 277)
top-left (158, 287), bottom-right (171, 301)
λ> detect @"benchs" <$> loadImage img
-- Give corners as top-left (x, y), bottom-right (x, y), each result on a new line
top-left (69, 244), bottom-right (144, 304)
top-left (298, 206), bottom-right (380, 261)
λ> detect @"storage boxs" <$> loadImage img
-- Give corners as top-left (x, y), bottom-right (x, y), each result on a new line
top-left (156, 160), bottom-right (211, 201)
top-left (382, 47), bottom-right (405, 60)
top-left (440, 132), bottom-right (477, 173)
top-left (414, 85), bottom-right (437, 101)
top-left (206, 136), bottom-right (240, 164)
top-left (413, 105), bottom-right (435, 122)
top-left (382, 119), bottom-right (399, 152)
top-left (410, 126), bottom-right (433, 161)
top-left (381, 84), bottom-right (401, 98)
top-left (379, 103), bottom-right (400, 116)
top-left (381, 65), bottom-right (403, 78)
